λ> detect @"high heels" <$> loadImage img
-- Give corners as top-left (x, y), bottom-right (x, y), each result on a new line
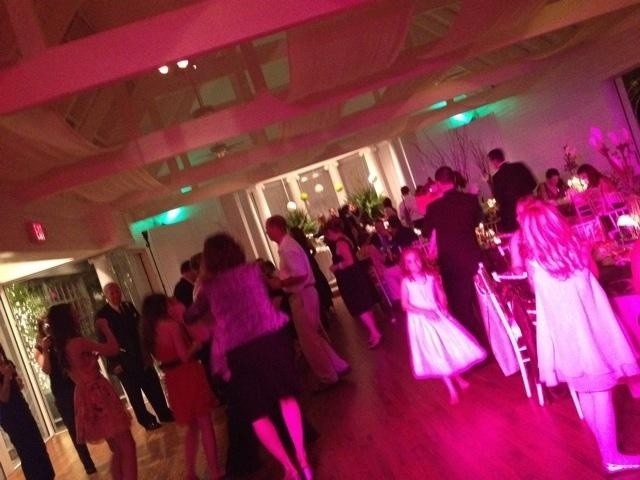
top-left (369, 333), bottom-right (375, 344)
top-left (370, 335), bottom-right (385, 349)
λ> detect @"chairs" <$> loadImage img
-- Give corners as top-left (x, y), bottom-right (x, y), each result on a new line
top-left (571, 220), bottom-right (596, 241)
top-left (366, 265), bottom-right (392, 306)
top-left (494, 272), bottom-right (584, 420)
top-left (589, 190), bottom-right (637, 244)
top-left (478, 263), bottom-right (532, 398)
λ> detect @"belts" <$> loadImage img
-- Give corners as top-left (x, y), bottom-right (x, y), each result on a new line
top-left (159, 359), bottom-right (186, 371)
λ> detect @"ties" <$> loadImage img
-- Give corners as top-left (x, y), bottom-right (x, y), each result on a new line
top-left (119, 306), bottom-right (127, 321)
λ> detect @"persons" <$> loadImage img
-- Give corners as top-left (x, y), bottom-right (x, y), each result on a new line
top-left (178, 259), bottom-right (230, 405)
top-left (1, 354), bottom-right (56, 479)
top-left (193, 231), bottom-right (314, 479)
top-left (401, 247), bottom-right (488, 404)
top-left (290, 225), bottom-right (337, 329)
top-left (34, 316), bottom-right (98, 476)
top-left (93, 282), bottom-right (174, 431)
top-left (574, 163), bottom-right (622, 215)
top-left (265, 215), bottom-right (350, 393)
top-left (139, 293), bottom-right (221, 479)
top-left (510, 196), bottom-right (546, 294)
top-left (521, 201), bottom-right (639, 473)
top-left (325, 217), bottom-right (383, 347)
top-left (489, 148), bottom-right (537, 232)
top-left (423, 165), bottom-right (492, 370)
top-left (47, 302), bottom-right (137, 479)
top-left (538, 168), bottom-right (565, 200)
top-left (335, 181), bottom-right (434, 264)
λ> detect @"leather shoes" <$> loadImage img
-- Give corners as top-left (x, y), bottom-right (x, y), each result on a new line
top-left (145, 421), bottom-right (160, 430)
top-left (315, 381), bottom-right (338, 393)
top-left (160, 412), bottom-right (177, 422)
top-left (337, 365), bottom-right (351, 377)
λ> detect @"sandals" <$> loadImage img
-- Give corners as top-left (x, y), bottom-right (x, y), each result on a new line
top-left (602, 455), bottom-right (640, 475)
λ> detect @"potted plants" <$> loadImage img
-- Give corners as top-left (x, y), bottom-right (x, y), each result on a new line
top-left (286, 209), bottom-right (316, 233)
top-left (345, 185), bottom-right (384, 219)
